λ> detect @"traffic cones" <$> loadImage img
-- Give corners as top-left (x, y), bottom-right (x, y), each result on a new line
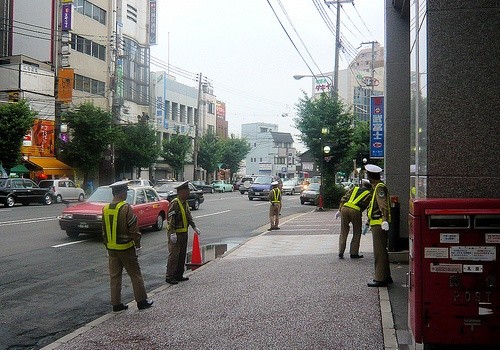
top-left (191, 231), bottom-right (204, 270)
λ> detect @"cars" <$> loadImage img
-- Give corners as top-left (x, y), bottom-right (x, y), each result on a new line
top-left (37, 180), bottom-right (87, 204)
top-left (0, 177), bottom-right (54, 208)
top-left (192, 180), bottom-right (216, 194)
top-left (153, 181), bottom-right (205, 209)
top-left (58, 185), bottom-right (170, 239)
top-left (210, 180), bottom-right (232, 193)
top-left (299, 183), bottom-right (323, 205)
top-left (282, 176), bottom-right (321, 195)
top-left (247, 176), bottom-right (283, 201)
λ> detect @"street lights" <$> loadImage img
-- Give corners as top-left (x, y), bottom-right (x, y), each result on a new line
top-left (293, 73), bottom-right (339, 186)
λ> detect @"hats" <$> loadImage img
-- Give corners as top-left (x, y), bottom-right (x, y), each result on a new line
top-left (362, 179), bottom-right (370, 186)
top-left (109, 181), bottom-right (133, 192)
top-left (174, 180), bottom-right (190, 190)
top-left (365, 164), bottom-right (382, 179)
top-left (270, 181), bottom-right (279, 186)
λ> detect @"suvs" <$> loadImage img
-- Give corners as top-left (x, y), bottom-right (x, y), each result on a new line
top-left (238, 176), bottom-right (256, 194)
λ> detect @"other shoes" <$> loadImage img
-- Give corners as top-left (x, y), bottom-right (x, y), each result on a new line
top-left (275, 227), bottom-right (280, 229)
top-left (268, 228), bottom-right (275, 230)
top-left (367, 277), bottom-right (393, 286)
top-left (113, 303), bottom-right (128, 312)
top-left (350, 254), bottom-right (363, 258)
top-left (176, 276), bottom-right (189, 281)
top-left (137, 299), bottom-right (153, 310)
top-left (338, 254), bottom-right (343, 259)
top-left (166, 278), bottom-right (178, 284)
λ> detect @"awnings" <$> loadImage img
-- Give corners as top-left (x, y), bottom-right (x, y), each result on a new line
top-left (0, 164), bottom-right (30, 173)
top-left (25, 159), bottom-right (74, 175)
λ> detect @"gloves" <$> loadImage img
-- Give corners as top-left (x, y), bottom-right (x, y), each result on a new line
top-left (381, 221), bottom-right (389, 231)
top-left (335, 211), bottom-right (341, 220)
top-left (194, 227), bottom-right (200, 235)
top-left (136, 247), bottom-right (142, 256)
top-left (170, 233), bottom-right (177, 244)
top-left (107, 249), bottom-right (109, 256)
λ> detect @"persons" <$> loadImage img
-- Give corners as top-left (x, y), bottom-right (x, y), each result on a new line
top-left (166, 180), bottom-right (201, 284)
top-left (365, 164), bottom-right (393, 286)
top-left (335, 179), bottom-right (372, 259)
top-left (102, 181), bottom-right (153, 311)
top-left (268, 182), bottom-right (281, 231)
